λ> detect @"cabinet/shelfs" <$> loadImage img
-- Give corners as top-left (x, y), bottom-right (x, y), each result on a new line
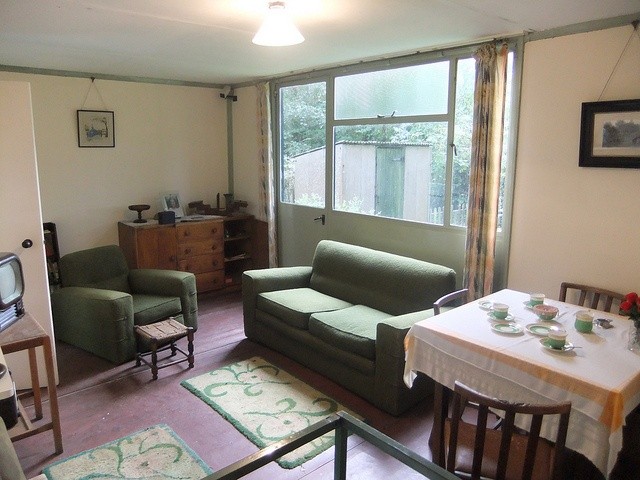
top-left (179, 218), bottom-right (224, 292)
top-left (118, 221), bottom-right (179, 274)
top-left (224, 210), bottom-right (255, 292)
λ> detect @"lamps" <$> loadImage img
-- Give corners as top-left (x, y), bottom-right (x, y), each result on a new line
top-left (251, 0), bottom-right (305, 47)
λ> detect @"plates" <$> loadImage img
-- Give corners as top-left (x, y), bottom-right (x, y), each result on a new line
top-left (527, 321), bottom-right (554, 337)
top-left (492, 322), bottom-right (523, 336)
top-left (539, 337), bottom-right (576, 353)
top-left (523, 301), bottom-right (547, 310)
top-left (479, 298), bottom-right (494, 308)
top-left (487, 311), bottom-right (517, 323)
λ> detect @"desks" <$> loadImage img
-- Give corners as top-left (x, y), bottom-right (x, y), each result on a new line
top-left (0, 311), bottom-right (64, 455)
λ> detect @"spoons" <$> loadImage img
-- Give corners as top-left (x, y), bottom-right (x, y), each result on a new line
top-left (558, 344), bottom-right (586, 352)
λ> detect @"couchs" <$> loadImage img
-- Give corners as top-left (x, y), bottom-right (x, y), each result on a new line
top-left (242, 239), bottom-right (459, 417)
top-left (52, 244), bottom-right (199, 363)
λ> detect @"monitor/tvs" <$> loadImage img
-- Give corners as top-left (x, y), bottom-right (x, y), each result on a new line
top-left (1, 252), bottom-right (26, 332)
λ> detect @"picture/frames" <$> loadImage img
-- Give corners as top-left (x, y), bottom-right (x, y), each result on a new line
top-left (77, 110), bottom-right (115, 148)
top-left (577, 98), bottom-right (640, 170)
top-left (162, 193), bottom-right (185, 218)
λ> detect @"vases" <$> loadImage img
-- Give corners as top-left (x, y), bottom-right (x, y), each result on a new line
top-left (627, 322), bottom-right (639, 350)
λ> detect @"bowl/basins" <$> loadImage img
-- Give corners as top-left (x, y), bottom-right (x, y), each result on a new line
top-left (533, 306), bottom-right (561, 321)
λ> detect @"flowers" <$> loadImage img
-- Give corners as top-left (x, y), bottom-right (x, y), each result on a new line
top-left (617, 291), bottom-right (640, 324)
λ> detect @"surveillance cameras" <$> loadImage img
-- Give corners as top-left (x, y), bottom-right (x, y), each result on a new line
top-left (220, 85), bottom-right (238, 100)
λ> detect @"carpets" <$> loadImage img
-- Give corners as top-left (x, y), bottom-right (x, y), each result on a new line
top-left (180, 354), bottom-right (369, 469)
top-left (42, 421), bottom-right (214, 480)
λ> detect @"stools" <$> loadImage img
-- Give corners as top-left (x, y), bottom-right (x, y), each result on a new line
top-left (133, 317), bottom-right (195, 380)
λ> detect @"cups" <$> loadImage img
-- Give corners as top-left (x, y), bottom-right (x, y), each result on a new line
top-left (491, 306), bottom-right (510, 318)
top-left (531, 294), bottom-right (547, 306)
top-left (549, 324), bottom-right (568, 347)
top-left (574, 308), bottom-right (594, 334)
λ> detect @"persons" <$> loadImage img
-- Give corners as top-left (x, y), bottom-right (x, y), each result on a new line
top-left (169, 199), bottom-right (182, 216)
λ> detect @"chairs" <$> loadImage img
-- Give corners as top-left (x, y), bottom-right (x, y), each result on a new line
top-left (447, 380), bottom-right (573, 480)
top-left (558, 282), bottom-right (627, 316)
